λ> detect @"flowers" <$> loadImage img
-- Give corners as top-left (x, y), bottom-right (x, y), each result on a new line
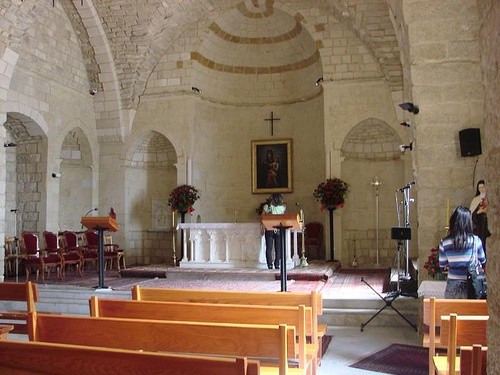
top-left (168, 184), bottom-right (200, 215)
top-left (423, 245), bottom-right (447, 279)
top-left (313, 177), bottom-right (351, 213)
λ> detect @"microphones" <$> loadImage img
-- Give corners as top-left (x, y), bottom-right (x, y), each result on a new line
top-left (81, 208), bottom-right (98, 229)
top-left (397, 181), bottom-right (415, 191)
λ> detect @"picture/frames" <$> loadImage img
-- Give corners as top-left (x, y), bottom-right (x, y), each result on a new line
top-left (251, 138), bottom-right (292, 194)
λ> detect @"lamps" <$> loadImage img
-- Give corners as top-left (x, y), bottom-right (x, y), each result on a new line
top-left (399, 141), bottom-right (412, 153)
top-left (315, 77), bottom-right (323, 86)
top-left (52, 173), bottom-right (62, 178)
top-left (192, 87), bottom-right (202, 94)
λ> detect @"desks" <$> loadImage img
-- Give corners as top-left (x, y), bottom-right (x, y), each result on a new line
top-left (176, 222), bottom-right (295, 270)
top-left (417, 281), bottom-right (446, 299)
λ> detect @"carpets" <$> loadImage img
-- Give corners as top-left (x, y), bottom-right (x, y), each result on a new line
top-left (348, 343), bottom-right (459, 375)
top-left (296, 335), bottom-right (332, 358)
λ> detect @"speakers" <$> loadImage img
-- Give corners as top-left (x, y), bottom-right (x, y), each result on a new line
top-left (458, 128), bottom-right (482, 156)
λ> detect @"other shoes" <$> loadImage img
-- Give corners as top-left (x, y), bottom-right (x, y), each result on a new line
top-left (274, 262), bottom-right (279, 269)
top-left (268, 264), bottom-right (273, 269)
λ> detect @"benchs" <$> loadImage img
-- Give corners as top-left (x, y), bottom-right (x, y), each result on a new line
top-left (0, 280), bottom-right (328, 375)
top-left (422, 296), bottom-right (489, 375)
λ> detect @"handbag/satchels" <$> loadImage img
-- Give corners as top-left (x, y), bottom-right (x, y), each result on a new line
top-left (466, 235), bottom-right (488, 299)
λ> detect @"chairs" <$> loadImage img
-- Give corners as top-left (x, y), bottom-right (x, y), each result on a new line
top-left (4, 229), bottom-right (125, 283)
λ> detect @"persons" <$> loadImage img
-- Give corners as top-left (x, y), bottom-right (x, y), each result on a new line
top-left (437, 206), bottom-right (487, 300)
top-left (469, 180), bottom-right (487, 257)
top-left (259, 193), bottom-right (284, 269)
